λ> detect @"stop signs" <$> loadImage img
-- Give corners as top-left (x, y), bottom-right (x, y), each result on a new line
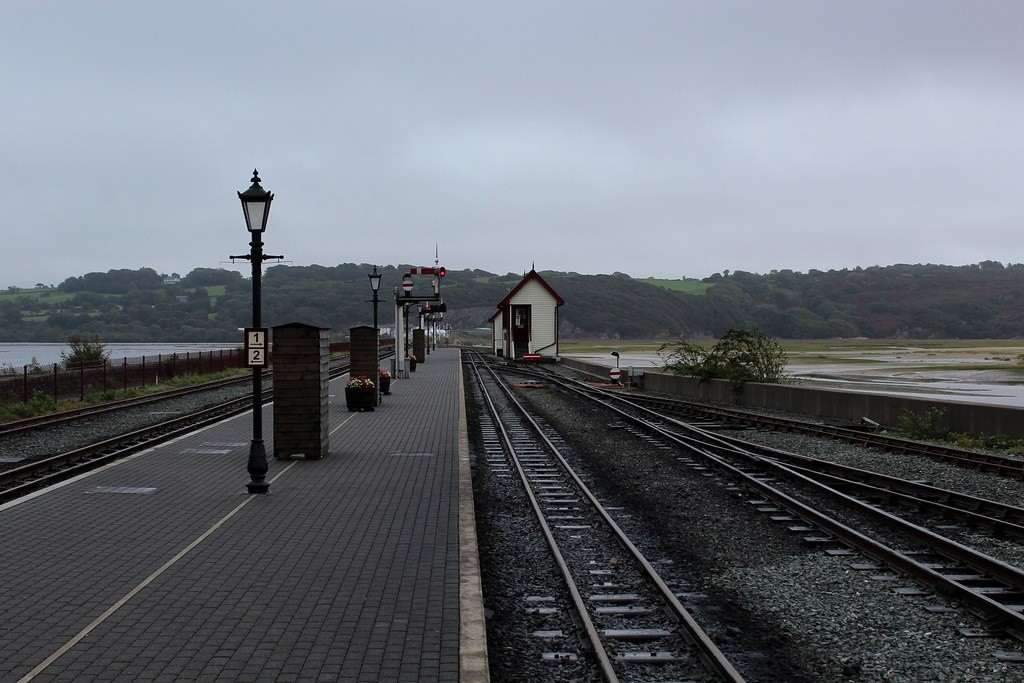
top-left (609, 368), bottom-right (621, 378)
top-left (402, 280), bottom-right (413, 291)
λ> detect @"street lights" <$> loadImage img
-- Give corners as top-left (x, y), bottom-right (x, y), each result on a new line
top-left (403, 273), bottom-right (412, 358)
top-left (230, 168), bottom-right (284, 493)
top-left (368, 265), bottom-right (386, 328)
top-left (417, 302), bottom-right (436, 355)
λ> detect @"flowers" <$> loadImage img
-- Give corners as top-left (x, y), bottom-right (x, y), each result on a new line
top-left (345, 376), bottom-right (375, 389)
top-left (380, 369), bottom-right (391, 377)
top-left (404, 354), bottom-right (416, 360)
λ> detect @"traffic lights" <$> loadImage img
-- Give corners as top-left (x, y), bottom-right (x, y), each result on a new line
top-left (440, 267), bottom-right (446, 278)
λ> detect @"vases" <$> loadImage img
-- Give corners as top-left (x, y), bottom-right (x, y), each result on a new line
top-left (345, 387), bottom-right (377, 408)
top-left (404, 359), bottom-right (417, 372)
top-left (379, 377), bottom-right (390, 393)
